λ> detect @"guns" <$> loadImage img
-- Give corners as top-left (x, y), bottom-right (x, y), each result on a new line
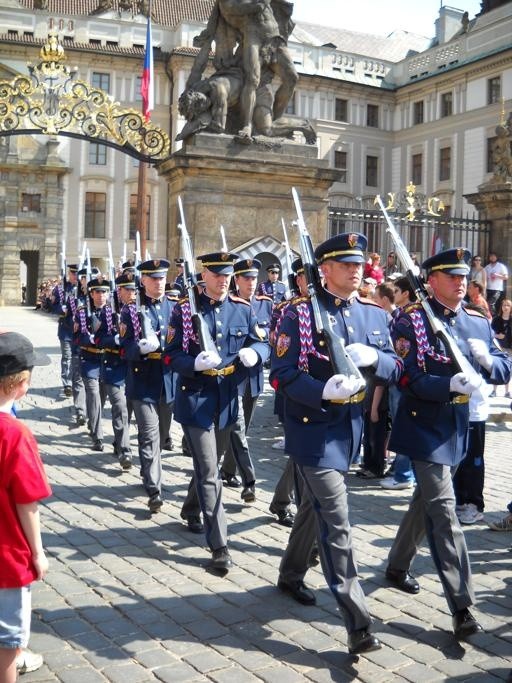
top-left (288, 185), bottom-right (367, 398)
top-left (280, 217), bottom-right (298, 295)
top-left (374, 194), bottom-right (479, 384)
top-left (175, 194), bottom-right (221, 364)
top-left (61, 239), bottom-right (127, 337)
top-left (135, 229), bottom-right (160, 351)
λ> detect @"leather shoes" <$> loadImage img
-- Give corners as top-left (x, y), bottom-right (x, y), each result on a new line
top-left (219, 466), bottom-right (242, 487)
top-left (385, 564), bottom-right (419, 593)
top-left (64, 385), bottom-right (192, 468)
top-left (148, 492), bottom-right (164, 512)
top-left (241, 484), bottom-right (256, 502)
top-left (269, 504), bottom-right (294, 527)
top-left (213, 545), bottom-right (232, 568)
top-left (452, 607), bottom-right (483, 639)
top-left (278, 572), bottom-right (316, 605)
top-left (181, 510), bottom-right (205, 532)
top-left (347, 626), bottom-right (381, 654)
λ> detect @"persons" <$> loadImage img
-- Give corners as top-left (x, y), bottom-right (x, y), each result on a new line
top-left (0, 330), bottom-right (46, 682)
top-left (269, 231), bottom-right (512, 654)
top-left (174, 0), bottom-right (315, 145)
top-left (38, 252), bottom-right (288, 569)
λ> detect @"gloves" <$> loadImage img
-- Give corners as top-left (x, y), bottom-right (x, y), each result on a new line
top-left (450, 370), bottom-right (482, 395)
top-left (194, 350), bottom-right (222, 372)
top-left (238, 346), bottom-right (260, 367)
top-left (322, 373), bottom-right (362, 400)
top-left (345, 342), bottom-right (378, 368)
top-left (138, 338), bottom-right (159, 356)
top-left (89, 333), bottom-right (96, 344)
top-left (114, 333), bottom-right (121, 344)
top-left (61, 304), bottom-right (68, 314)
top-left (466, 337), bottom-right (494, 370)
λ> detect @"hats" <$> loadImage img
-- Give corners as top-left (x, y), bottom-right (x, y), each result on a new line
top-left (0, 331), bottom-right (52, 376)
top-left (314, 231), bottom-right (368, 262)
top-left (421, 247), bottom-right (472, 275)
top-left (67, 257), bottom-right (187, 291)
top-left (266, 263), bottom-right (281, 272)
top-left (196, 252), bottom-right (239, 274)
top-left (234, 258), bottom-right (262, 276)
top-left (291, 257), bottom-right (324, 277)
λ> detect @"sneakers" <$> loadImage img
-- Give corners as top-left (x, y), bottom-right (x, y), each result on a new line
top-left (356, 468), bottom-right (366, 476)
top-left (361, 470), bottom-right (375, 478)
top-left (381, 479), bottom-right (413, 489)
top-left (488, 511), bottom-right (512, 531)
top-left (16, 648), bottom-right (45, 673)
top-left (272, 439), bottom-right (285, 449)
top-left (459, 502), bottom-right (484, 523)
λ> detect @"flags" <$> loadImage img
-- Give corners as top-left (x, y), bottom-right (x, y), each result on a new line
top-left (139, 7), bottom-right (155, 119)
